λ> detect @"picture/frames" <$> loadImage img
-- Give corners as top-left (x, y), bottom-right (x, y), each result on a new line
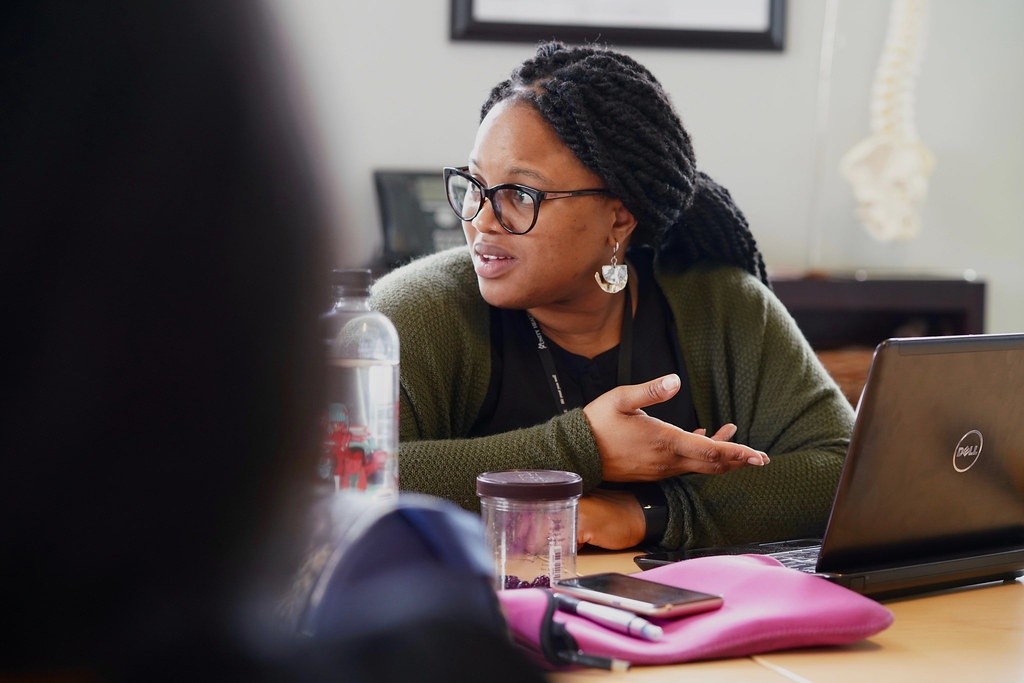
top-left (447, 0), bottom-right (788, 55)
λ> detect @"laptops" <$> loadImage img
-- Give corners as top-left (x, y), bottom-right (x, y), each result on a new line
top-left (632, 333), bottom-right (1024, 596)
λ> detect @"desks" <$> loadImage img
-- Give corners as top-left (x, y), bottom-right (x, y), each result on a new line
top-left (496, 546), bottom-right (1023, 683)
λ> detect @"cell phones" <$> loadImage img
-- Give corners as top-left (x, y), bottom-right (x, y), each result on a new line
top-left (555, 572), bottom-right (723, 618)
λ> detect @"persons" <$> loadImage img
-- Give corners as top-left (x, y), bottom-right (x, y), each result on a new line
top-left (0, 2), bottom-right (554, 682)
top-left (315, 37), bottom-right (858, 557)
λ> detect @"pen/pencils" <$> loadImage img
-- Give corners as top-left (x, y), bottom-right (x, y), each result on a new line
top-left (549, 592), bottom-right (667, 647)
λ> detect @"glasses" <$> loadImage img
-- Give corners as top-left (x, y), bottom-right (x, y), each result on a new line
top-left (442, 166), bottom-right (613, 235)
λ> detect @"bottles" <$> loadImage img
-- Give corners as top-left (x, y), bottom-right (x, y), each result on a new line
top-left (317, 270), bottom-right (402, 498)
top-left (473, 467), bottom-right (583, 595)
